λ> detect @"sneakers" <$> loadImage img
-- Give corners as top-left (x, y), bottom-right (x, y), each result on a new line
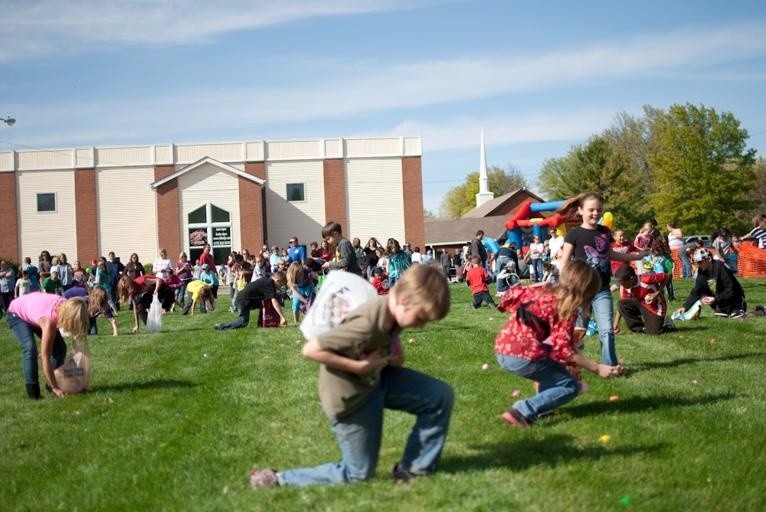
top-left (502, 408), bottom-right (533, 428)
top-left (250, 468), bottom-right (278, 491)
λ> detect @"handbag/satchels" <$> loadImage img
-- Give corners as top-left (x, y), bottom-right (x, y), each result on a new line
top-left (262, 298), bottom-right (281, 326)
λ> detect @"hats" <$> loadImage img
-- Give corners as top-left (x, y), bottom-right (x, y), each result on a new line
top-left (689, 248), bottom-right (710, 265)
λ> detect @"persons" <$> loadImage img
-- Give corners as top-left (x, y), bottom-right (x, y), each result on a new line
top-left (249, 263), bottom-right (456, 491)
top-left (123, 253), bottom-right (145, 310)
top-left (4, 250), bottom-right (125, 316)
top-left (62, 286), bottom-right (119, 336)
top-left (556, 194), bottom-right (651, 377)
top-left (226, 245), bottom-right (291, 313)
top-left (466, 255), bottom-right (496, 309)
top-left (285, 221), bottom-right (434, 326)
top-left (492, 259), bottom-right (624, 432)
top-left (219, 264), bottom-right (229, 288)
top-left (609, 214), bottom-right (766, 335)
top-left (440, 228), bottom-right (565, 297)
top-left (6, 292), bottom-right (89, 400)
top-left (152, 243), bottom-right (220, 315)
top-left (118, 274), bottom-right (175, 334)
top-left (214, 271), bottom-right (287, 331)
